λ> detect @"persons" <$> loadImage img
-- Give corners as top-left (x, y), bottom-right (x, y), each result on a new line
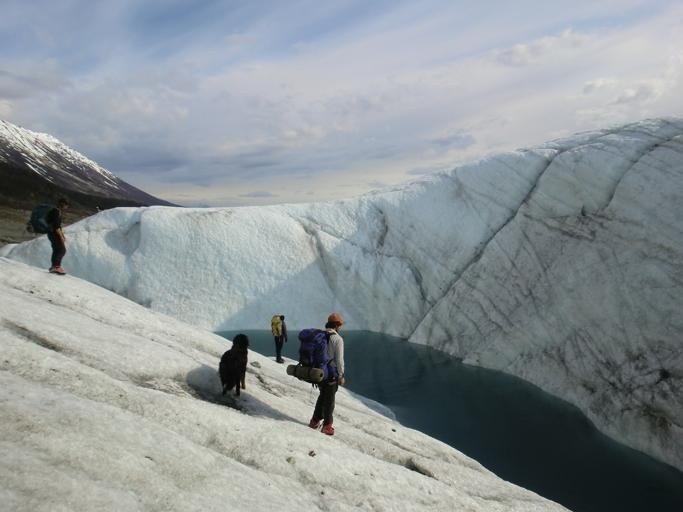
top-left (307, 313), bottom-right (345, 435)
top-left (46, 197), bottom-right (70, 274)
top-left (275, 315), bottom-right (288, 363)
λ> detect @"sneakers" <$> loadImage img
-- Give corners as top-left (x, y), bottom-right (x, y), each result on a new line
top-left (309, 419), bottom-right (335, 435)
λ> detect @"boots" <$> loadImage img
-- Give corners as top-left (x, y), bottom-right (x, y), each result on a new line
top-left (49, 261), bottom-right (66, 275)
top-left (276, 358), bottom-right (284, 363)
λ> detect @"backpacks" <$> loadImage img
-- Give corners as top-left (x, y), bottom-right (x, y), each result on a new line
top-left (27, 202), bottom-right (61, 233)
top-left (271, 316), bottom-right (282, 336)
top-left (298, 328), bottom-right (336, 388)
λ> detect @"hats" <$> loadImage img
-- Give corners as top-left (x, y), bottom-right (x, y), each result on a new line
top-left (328, 313), bottom-right (345, 324)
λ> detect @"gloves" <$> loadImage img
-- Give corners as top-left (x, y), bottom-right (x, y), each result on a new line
top-left (285, 338), bottom-right (287, 343)
